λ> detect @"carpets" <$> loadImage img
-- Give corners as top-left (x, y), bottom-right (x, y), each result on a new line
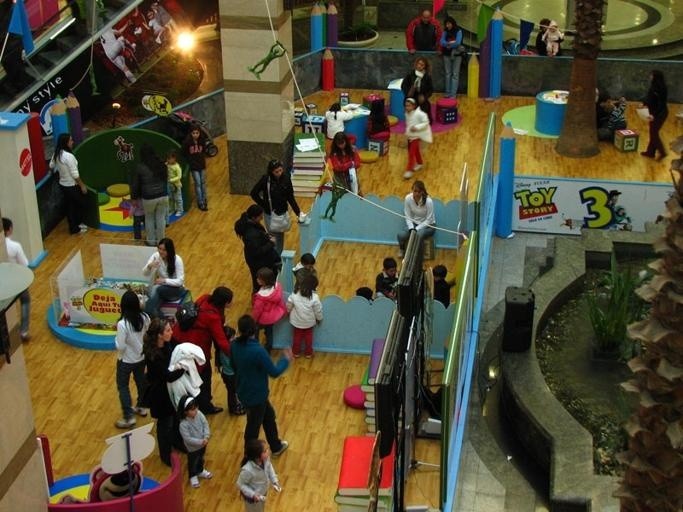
top-left (383, 103), bottom-right (462, 134)
top-left (502, 104), bottom-right (559, 139)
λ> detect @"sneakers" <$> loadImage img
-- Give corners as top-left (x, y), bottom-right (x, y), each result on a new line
top-left (78, 229), bottom-right (88, 233)
top-left (235, 401), bottom-right (244, 415)
top-left (397, 249), bottom-right (404, 258)
top-left (132, 408), bottom-right (148, 417)
top-left (175, 210), bottom-right (183, 216)
top-left (190, 476), bottom-right (200, 487)
top-left (656, 152), bottom-right (666, 160)
top-left (403, 170), bottom-right (415, 179)
top-left (272, 440), bottom-right (289, 459)
top-left (294, 352), bottom-right (300, 357)
top-left (79, 224), bottom-right (87, 228)
top-left (641, 151), bottom-right (655, 158)
top-left (200, 202), bottom-right (208, 211)
top-left (21, 332), bottom-right (30, 340)
top-left (414, 165), bottom-right (424, 170)
top-left (196, 469), bottom-right (213, 479)
top-left (116, 418), bottom-right (137, 429)
top-left (304, 353), bottom-right (312, 358)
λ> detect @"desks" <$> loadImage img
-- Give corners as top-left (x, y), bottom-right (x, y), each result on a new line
top-left (534, 90), bottom-right (570, 136)
top-left (389, 78), bottom-right (406, 120)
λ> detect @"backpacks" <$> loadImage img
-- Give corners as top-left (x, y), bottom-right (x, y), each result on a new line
top-left (176, 301), bottom-right (216, 333)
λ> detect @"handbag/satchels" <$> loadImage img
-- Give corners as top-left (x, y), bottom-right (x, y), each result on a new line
top-left (269, 208), bottom-right (292, 234)
top-left (347, 162), bottom-right (359, 195)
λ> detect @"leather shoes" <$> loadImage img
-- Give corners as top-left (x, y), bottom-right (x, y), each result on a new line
top-left (203, 407), bottom-right (223, 414)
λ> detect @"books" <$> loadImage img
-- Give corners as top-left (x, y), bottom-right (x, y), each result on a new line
top-left (333, 337), bottom-right (404, 511)
top-left (289, 132), bottom-right (326, 198)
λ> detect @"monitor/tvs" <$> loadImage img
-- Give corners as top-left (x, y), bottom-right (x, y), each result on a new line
top-left (374, 312), bottom-right (406, 458)
top-left (394, 230), bottom-right (425, 311)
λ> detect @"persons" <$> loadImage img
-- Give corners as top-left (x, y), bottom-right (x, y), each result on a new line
top-left (251, 159), bottom-right (299, 256)
top-left (596, 93), bottom-right (625, 141)
top-left (536, 18), bottom-right (564, 55)
top-left (99, 0), bottom-right (178, 83)
top-left (325, 103), bottom-right (352, 140)
top-left (608, 190), bottom-right (632, 228)
top-left (164, 150), bottom-right (184, 216)
top-left (181, 126), bottom-right (207, 211)
top-left (401, 7), bottom-right (465, 180)
top-left (2, 217), bottom-right (30, 340)
top-left (129, 197), bottom-right (145, 240)
top-left (375, 257), bottom-right (399, 306)
top-left (357, 287), bottom-right (374, 305)
top-left (234, 205), bottom-right (278, 294)
top-left (542, 20), bottom-right (564, 56)
top-left (432, 264), bottom-right (450, 309)
top-left (328, 132), bottom-right (361, 193)
top-left (235, 439), bottom-right (279, 512)
top-left (116, 237), bottom-right (292, 489)
top-left (130, 144), bottom-right (169, 247)
top-left (639, 70), bottom-right (668, 161)
top-left (49, 133), bottom-right (88, 235)
top-left (250, 252), bottom-right (323, 359)
top-left (397, 181), bottom-right (436, 259)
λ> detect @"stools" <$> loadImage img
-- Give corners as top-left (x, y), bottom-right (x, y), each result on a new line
top-left (295, 92), bottom-right (390, 156)
top-left (423, 236), bottom-right (434, 260)
top-left (436, 97), bottom-right (458, 124)
top-left (614, 129), bottom-right (640, 152)
top-left (159, 290), bottom-right (192, 323)
